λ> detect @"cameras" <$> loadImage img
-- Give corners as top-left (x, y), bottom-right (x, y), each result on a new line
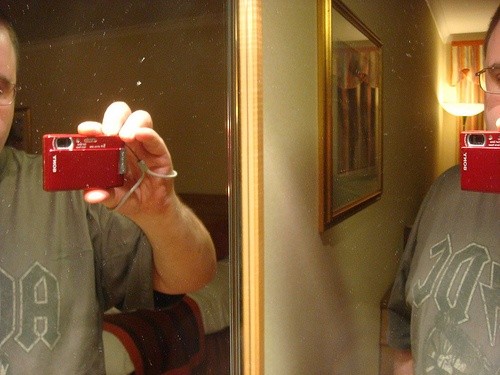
top-left (42, 134), bottom-right (128, 192)
top-left (460, 130), bottom-right (500, 194)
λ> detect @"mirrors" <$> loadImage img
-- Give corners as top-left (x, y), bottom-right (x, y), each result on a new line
top-left (0, 0), bottom-right (265, 375)
top-left (316, 0), bottom-right (383, 234)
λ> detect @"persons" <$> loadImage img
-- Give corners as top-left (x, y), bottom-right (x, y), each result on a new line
top-left (387, 4), bottom-right (500, 375)
top-left (0, 14), bottom-right (218, 375)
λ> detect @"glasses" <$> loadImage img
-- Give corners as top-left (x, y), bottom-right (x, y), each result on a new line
top-left (0, 82), bottom-right (23, 106)
top-left (475, 65), bottom-right (500, 93)
